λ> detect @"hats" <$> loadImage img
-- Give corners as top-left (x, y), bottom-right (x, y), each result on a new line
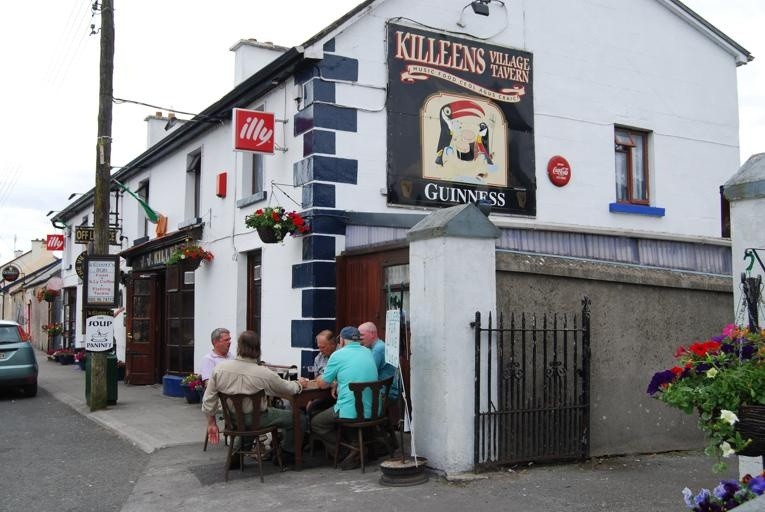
top-left (338, 326), bottom-right (365, 341)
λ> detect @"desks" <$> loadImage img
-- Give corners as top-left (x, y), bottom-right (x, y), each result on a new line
top-left (266, 380), bottom-right (336, 470)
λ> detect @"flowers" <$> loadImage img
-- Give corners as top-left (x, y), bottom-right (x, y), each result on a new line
top-left (179, 374), bottom-right (205, 395)
top-left (35, 287), bottom-right (59, 303)
top-left (164, 242), bottom-right (215, 265)
top-left (245, 205), bottom-right (311, 244)
top-left (647, 322), bottom-right (765, 475)
top-left (39, 320), bottom-right (85, 365)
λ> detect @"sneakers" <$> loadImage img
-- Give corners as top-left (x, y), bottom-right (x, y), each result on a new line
top-left (340, 442), bottom-right (372, 470)
top-left (250, 442), bottom-right (292, 466)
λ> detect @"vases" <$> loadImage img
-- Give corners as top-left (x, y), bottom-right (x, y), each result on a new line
top-left (183, 386), bottom-right (203, 403)
top-left (177, 258), bottom-right (202, 272)
top-left (45, 297), bottom-right (55, 302)
top-left (255, 227), bottom-right (287, 243)
top-left (714, 406), bottom-right (764, 456)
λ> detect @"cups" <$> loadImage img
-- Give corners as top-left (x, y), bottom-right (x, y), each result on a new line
top-left (307, 365), bottom-right (315, 381)
top-left (276, 367), bottom-right (298, 382)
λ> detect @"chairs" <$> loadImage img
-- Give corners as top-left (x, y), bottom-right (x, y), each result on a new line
top-left (217, 389), bottom-right (284, 483)
top-left (332, 375), bottom-right (396, 473)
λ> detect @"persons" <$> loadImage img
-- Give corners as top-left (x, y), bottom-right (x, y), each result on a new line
top-left (113, 307), bottom-right (126, 353)
top-left (201, 328), bottom-right (279, 454)
top-left (204, 332), bottom-right (309, 470)
top-left (305, 323), bottom-right (401, 470)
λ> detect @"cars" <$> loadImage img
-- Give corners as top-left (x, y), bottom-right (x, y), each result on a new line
top-left (0, 319), bottom-right (38, 398)
